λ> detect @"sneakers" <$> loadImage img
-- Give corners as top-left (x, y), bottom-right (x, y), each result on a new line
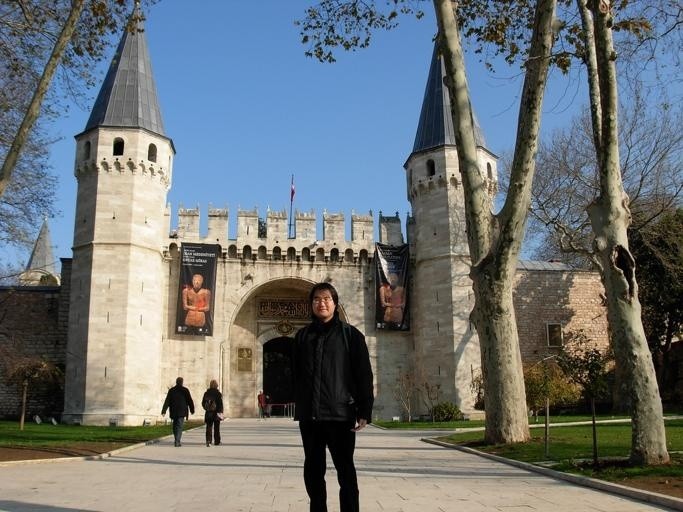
top-left (175, 441), bottom-right (181, 447)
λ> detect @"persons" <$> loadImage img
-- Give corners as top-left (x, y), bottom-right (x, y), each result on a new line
top-left (264, 394), bottom-right (271, 404)
top-left (181, 274), bottom-right (211, 327)
top-left (380, 273), bottom-right (406, 323)
top-left (256, 391), bottom-right (265, 419)
top-left (291, 282), bottom-right (375, 512)
top-left (201, 379), bottom-right (224, 447)
top-left (160, 377), bottom-right (194, 447)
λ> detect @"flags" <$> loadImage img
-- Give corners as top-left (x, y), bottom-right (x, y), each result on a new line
top-left (290, 176), bottom-right (296, 202)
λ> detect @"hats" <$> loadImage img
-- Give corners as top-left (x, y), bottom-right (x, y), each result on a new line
top-left (210, 380), bottom-right (218, 388)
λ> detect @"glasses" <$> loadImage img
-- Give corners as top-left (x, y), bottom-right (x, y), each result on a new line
top-left (312, 297), bottom-right (333, 304)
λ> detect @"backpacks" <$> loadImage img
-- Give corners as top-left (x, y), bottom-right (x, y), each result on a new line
top-left (204, 392), bottom-right (220, 412)
